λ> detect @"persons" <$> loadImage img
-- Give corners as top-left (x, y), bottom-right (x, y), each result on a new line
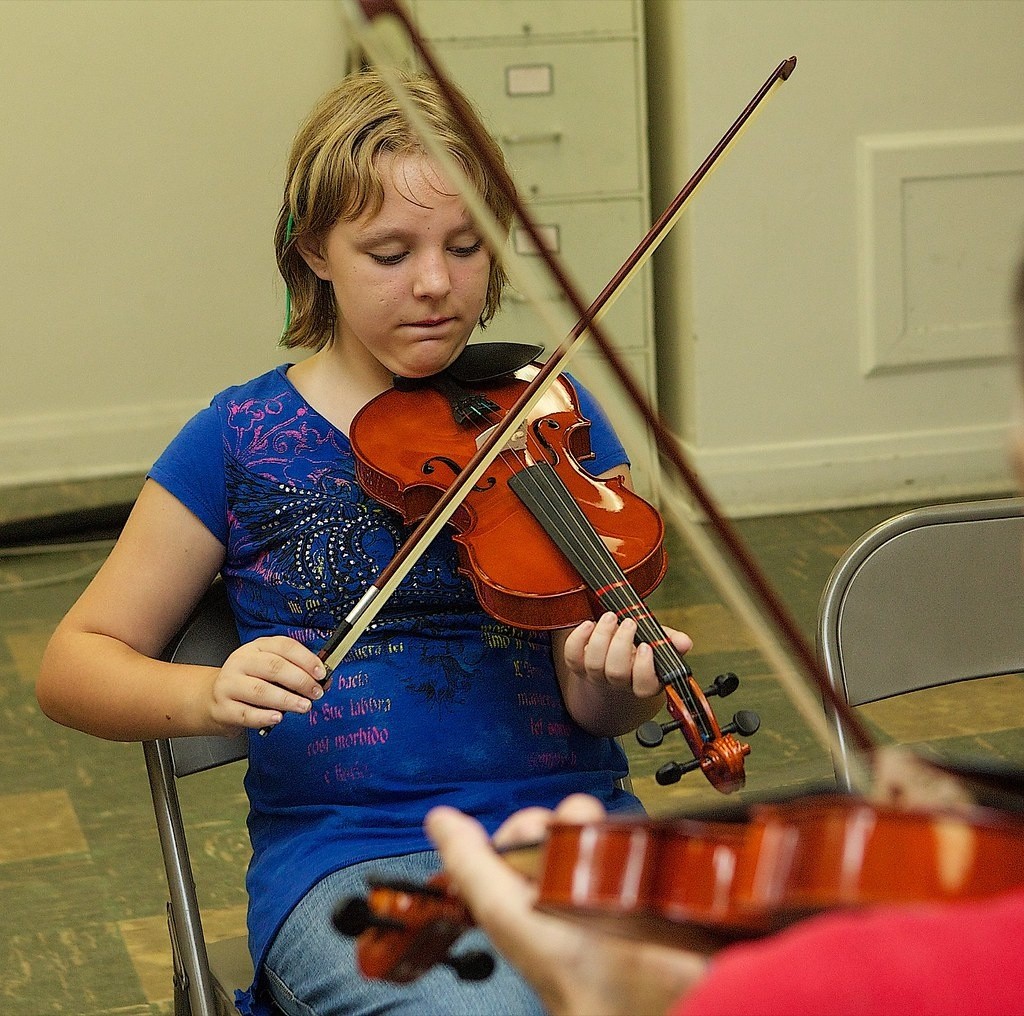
top-left (423, 793), bottom-right (1024, 1016)
top-left (36, 62), bottom-right (693, 1016)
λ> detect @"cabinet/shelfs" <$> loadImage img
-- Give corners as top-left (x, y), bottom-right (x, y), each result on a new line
top-left (367, 0), bottom-right (662, 513)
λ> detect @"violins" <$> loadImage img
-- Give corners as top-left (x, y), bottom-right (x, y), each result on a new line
top-left (327, 790), bottom-right (1024, 986)
top-left (347, 356), bottom-right (761, 796)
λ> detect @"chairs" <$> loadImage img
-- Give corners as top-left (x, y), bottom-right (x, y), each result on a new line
top-left (815, 498), bottom-right (1023, 794)
top-left (142, 579), bottom-right (254, 1016)
